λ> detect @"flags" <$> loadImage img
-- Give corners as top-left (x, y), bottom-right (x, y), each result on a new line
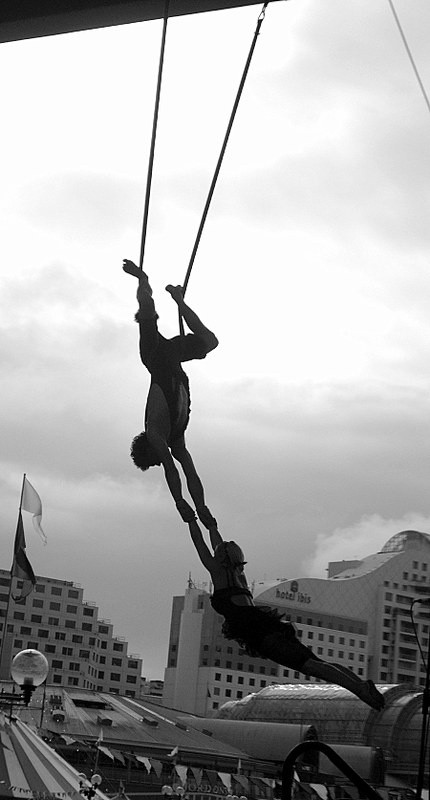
top-left (21, 478), bottom-right (48, 545)
top-left (10, 513), bottom-right (37, 602)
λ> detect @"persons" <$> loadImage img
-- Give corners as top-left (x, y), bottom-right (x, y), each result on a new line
top-left (120, 260), bottom-right (220, 530)
top-left (180, 498), bottom-right (386, 713)
